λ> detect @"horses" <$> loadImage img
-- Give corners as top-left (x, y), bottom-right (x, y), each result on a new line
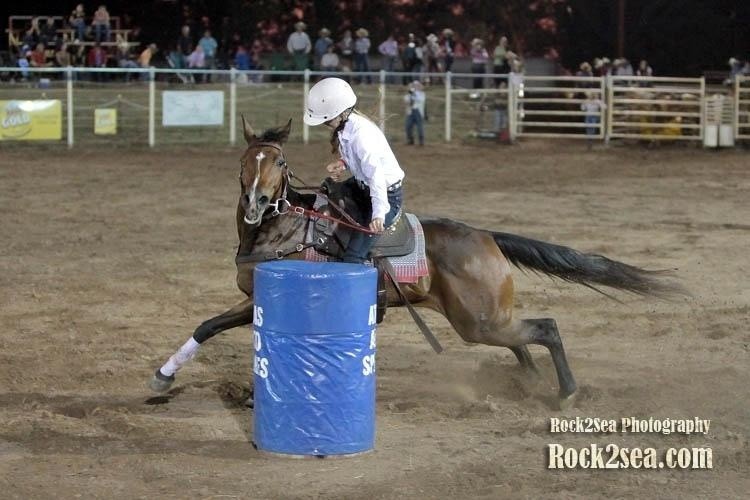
top-left (144, 115), bottom-right (690, 415)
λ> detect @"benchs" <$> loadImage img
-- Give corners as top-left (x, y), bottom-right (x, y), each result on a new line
top-left (9, 12), bottom-right (144, 69)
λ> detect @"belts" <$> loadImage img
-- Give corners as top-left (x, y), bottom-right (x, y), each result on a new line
top-left (356, 181), bottom-right (403, 193)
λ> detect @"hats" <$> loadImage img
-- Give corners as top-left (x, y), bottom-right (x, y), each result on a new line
top-left (407, 80), bottom-right (425, 93)
top-left (579, 56), bottom-right (628, 72)
top-left (318, 28), bottom-right (331, 36)
top-left (355, 28), bottom-right (368, 37)
top-left (442, 29), bottom-right (455, 35)
top-left (293, 22), bottom-right (306, 30)
top-left (427, 34), bottom-right (438, 43)
top-left (471, 38), bottom-right (484, 47)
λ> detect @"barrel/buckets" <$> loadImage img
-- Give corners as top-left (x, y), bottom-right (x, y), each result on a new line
top-left (251, 259), bottom-right (377, 458)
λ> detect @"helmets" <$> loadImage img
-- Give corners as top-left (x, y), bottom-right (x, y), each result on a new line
top-left (303, 76), bottom-right (358, 127)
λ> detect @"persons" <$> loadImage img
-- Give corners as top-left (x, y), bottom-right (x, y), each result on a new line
top-left (303, 77), bottom-right (405, 262)
top-left (9, 3), bottom-right (750, 146)
